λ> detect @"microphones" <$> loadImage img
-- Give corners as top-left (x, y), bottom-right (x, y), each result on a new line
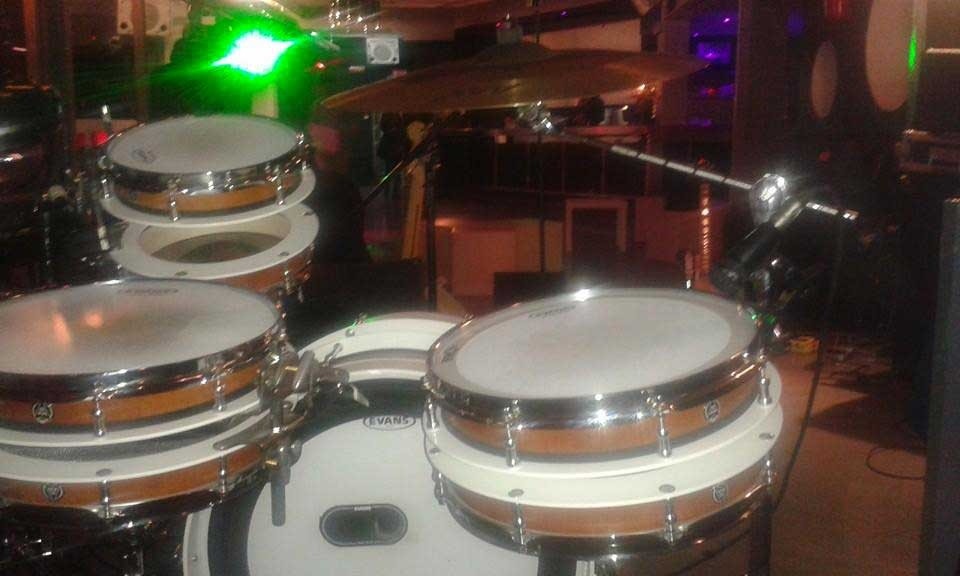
top-left (706, 183), bottom-right (823, 294)
top-left (90, 193), bottom-right (113, 254)
top-left (538, 95), bottom-right (604, 135)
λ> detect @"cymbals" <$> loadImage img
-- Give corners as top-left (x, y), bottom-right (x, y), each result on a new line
top-left (318, 42), bottom-right (690, 115)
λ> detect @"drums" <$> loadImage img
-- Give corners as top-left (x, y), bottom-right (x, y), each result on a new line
top-left (0, 280), bottom-right (310, 515)
top-left (420, 286), bottom-right (784, 541)
top-left (98, 111), bottom-right (321, 297)
top-left (182, 310), bottom-right (597, 576)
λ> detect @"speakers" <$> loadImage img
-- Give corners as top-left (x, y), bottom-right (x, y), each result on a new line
top-left (365, 35), bottom-right (402, 65)
